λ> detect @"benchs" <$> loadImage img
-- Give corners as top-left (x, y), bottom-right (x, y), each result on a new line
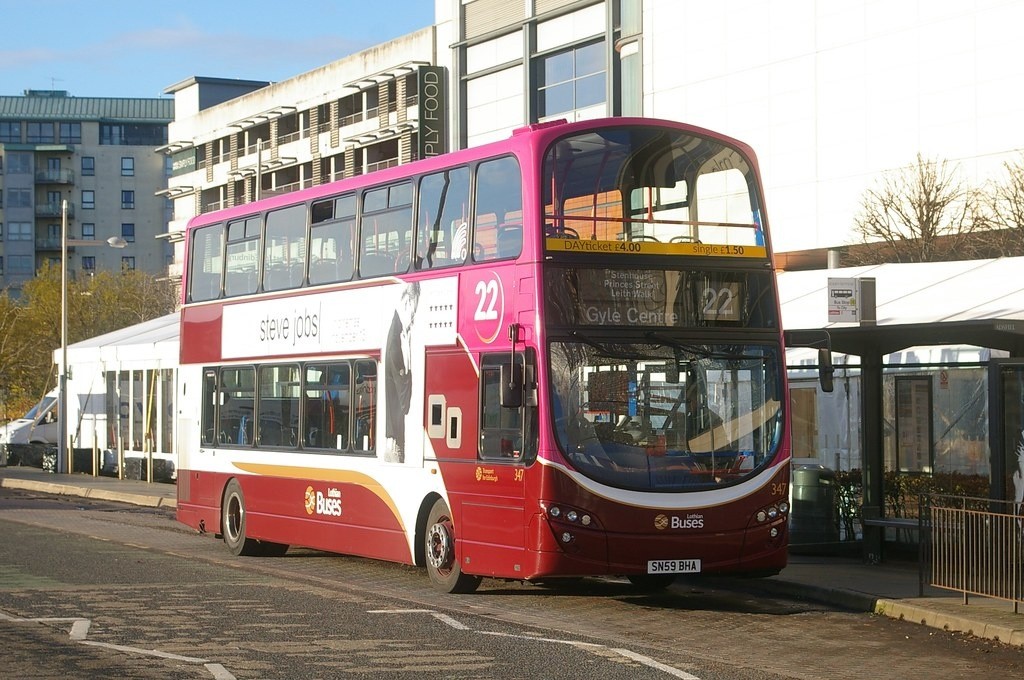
top-left (862, 516), bottom-right (968, 564)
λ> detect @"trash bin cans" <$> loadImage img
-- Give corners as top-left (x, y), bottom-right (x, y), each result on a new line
top-left (792, 465), bottom-right (841, 544)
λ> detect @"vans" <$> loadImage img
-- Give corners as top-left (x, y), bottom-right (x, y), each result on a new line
top-left (0, 385), bottom-right (60, 468)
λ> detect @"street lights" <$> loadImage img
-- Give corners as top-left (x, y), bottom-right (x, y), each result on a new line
top-left (57, 198), bottom-right (130, 475)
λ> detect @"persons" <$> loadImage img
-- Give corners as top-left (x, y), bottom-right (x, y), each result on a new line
top-left (384, 281), bottom-right (421, 463)
top-left (678, 385), bottom-right (731, 450)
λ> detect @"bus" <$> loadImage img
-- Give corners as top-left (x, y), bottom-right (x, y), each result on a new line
top-left (173, 114), bottom-right (835, 594)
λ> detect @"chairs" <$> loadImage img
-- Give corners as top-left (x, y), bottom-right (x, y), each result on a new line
top-left (220, 409), bottom-right (240, 443)
top-left (209, 221), bottom-right (704, 299)
top-left (357, 409), bottom-right (375, 448)
top-left (237, 415), bottom-right (283, 444)
